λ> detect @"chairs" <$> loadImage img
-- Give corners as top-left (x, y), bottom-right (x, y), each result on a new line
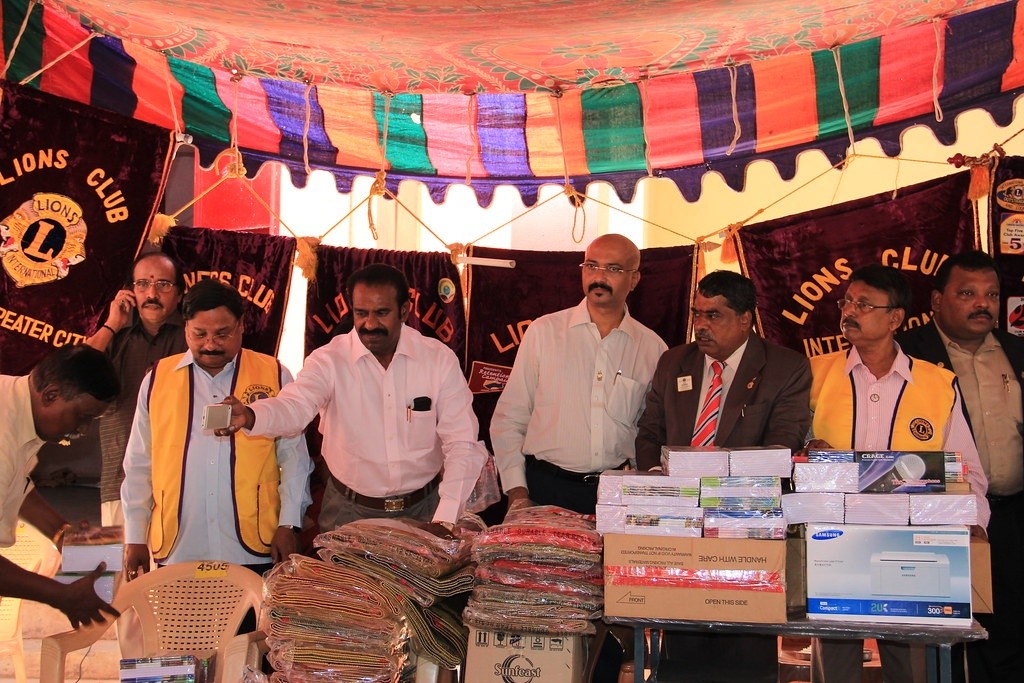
top-left (40, 559), bottom-right (275, 683)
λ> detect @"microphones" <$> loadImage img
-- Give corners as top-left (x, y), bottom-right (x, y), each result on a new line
top-left (860, 456), bottom-right (927, 495)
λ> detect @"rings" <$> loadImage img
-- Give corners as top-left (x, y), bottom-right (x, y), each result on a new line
top-left (128, 571), bottom-right (138, 574)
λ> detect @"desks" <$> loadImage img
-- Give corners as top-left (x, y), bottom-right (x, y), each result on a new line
top-left (602, 609), bottom-right (988, 683)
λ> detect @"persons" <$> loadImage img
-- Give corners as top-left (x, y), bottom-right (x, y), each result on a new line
top-left (793, 266), bottom-right (990, 683)
top-left (120, 280), bottom-right (315, 635)
top-left (0, 344), bottom-right (120, 629)
top-left (894, 251), bottom-right (1024, 683)
top-left (215, 263), bottom-right (489, 527)
top-left (85, 252), bottom-right (189, 527)
top-left (633, 271), bottom-right (814, 683)
top-left (489, 235), bottom-right (669, 512)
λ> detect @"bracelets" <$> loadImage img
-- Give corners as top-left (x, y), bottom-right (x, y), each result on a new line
top-left (103, 325), bottom-right (116, 335)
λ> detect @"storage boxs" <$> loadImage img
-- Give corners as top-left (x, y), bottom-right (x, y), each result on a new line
top-left (451, 432), bottom-right (994, 682)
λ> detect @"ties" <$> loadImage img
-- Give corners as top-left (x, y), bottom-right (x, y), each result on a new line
top-left (689, 360), bottom-right (729, 451)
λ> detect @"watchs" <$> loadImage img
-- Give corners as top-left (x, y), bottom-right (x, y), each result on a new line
top-left (283, 525), bottom-right (301, 534)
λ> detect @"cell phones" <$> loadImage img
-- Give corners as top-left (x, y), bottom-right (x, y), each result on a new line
top-left (202, 405), bottom-right (232, 429)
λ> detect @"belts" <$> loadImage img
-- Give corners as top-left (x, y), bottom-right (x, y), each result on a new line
top-left (526, 455), bottom-right (631, 487)
top-left (329, 474), bottom-right (441, 513)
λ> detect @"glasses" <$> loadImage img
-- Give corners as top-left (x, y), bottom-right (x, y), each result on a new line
top-left (835, 297), bottom-right (902, 314)
top-left (189, 321), bottom-right (239, 344)
top-left (580, 262), bottom-right (637, 280)
top-left (132, 279), bottom-right (178, 292)
